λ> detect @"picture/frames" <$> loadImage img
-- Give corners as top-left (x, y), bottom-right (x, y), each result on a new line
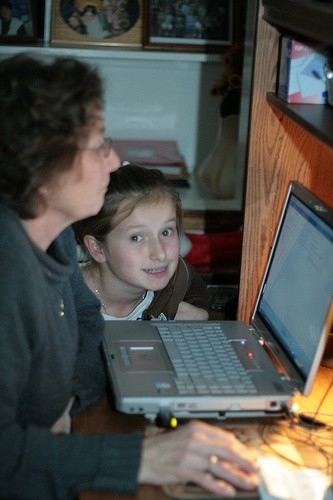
top-left (0, 0), bottom-right (237, 54)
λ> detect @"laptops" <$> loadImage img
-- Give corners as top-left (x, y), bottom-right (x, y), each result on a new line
top-left (100, 180), bottom-right (333, 415)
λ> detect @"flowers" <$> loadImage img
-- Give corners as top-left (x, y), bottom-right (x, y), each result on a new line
top-left (209, 52), bottom-right (243, 116)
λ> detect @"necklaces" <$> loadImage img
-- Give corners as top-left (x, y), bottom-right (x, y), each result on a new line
top-left (91, 271), bottom-right (109, 316)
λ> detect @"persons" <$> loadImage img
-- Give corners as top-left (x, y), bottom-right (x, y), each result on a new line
top-left (69, 165), bottom-right (212, 322)
top-left (0, 0), bottom-right (228, 38)
top-left (0, 48), bottom-right (260, 500)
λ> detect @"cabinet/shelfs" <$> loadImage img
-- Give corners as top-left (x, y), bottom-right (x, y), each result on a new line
top-left (68, 1), bottom-right (333, 500)
top-left (1, 40), bottom-right (252, 231)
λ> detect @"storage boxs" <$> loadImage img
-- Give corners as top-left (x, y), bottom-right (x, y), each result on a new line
top-left (276, 34), bottom-right (333, 105)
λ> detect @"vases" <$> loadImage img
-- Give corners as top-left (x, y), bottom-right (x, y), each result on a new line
top-left (198, 113), bottom-right (238, 199)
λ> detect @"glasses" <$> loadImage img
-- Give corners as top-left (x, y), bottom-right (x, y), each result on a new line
top-left (71, 136), bottom-right (112, 158)
